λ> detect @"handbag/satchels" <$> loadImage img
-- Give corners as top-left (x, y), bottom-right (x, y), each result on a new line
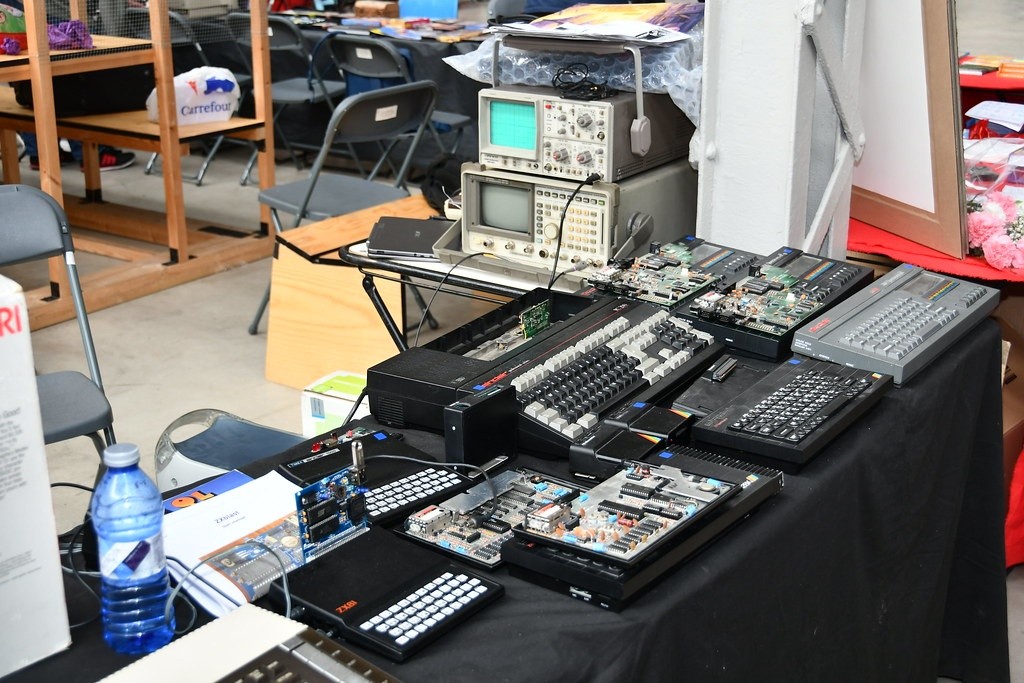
top-left (146, 66), bottom-right (241, 127)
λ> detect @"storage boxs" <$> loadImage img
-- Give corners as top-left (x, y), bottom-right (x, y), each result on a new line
top-left (301, 372), bottom-right (371, 438)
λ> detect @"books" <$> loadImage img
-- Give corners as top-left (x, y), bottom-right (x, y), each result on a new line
top-left (958, 56), bottom-right (1013, 76)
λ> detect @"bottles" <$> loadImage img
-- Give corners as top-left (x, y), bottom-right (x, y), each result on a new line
top-left (92, 443), bottom-right (175, 654)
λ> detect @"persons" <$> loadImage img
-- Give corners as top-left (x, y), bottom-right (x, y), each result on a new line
top-left (0, 0), bottom-right (145, 173)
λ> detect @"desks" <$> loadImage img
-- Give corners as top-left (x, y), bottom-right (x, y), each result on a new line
top-left (339, 238), bottom-right (575, 352)
top-left (171, 14), bottom-right (492, 180)
top-left (0, 318), bottom-right (1012, 683)
top-left (958, 56), bottom-right (1024, 103)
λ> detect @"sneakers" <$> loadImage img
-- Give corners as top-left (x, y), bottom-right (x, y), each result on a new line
top-left (29, 150), bottom-right (73, 169)
top-left (79, 150), bottom-right (136, 171)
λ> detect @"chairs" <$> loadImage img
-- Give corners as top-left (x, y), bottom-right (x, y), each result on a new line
top-left (249, 81), bottom-right (438, 335)
top-left (1, 184), bottom-right (117, 461)
top-left (125, 7), bottom-right (258, 186)
top-left (225, 12), bottom-right (368, 186)
top-left (325, 34), bottom-right (471, 195)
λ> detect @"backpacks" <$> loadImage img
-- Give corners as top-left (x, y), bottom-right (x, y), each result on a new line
top-left (422, 154), bottom-right (472, 216)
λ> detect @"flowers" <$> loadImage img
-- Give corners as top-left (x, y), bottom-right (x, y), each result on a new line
top-left (967, 191), bottom-right (1024, 269)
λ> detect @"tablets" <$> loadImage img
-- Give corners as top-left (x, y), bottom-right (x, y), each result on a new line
top-left (367, 216), bottom-right (456, 261)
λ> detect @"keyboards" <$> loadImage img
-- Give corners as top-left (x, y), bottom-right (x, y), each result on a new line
top-left (364, 468), bottom-right (462, 517)
top-left (361, 571), bottom-right (487, 646)
top-left (495, 303), bottom-right (724, 449)
top-left (691, 356), bottom-right (893, 464)
top-left (789, 263), bottom-right (1000, 388)
